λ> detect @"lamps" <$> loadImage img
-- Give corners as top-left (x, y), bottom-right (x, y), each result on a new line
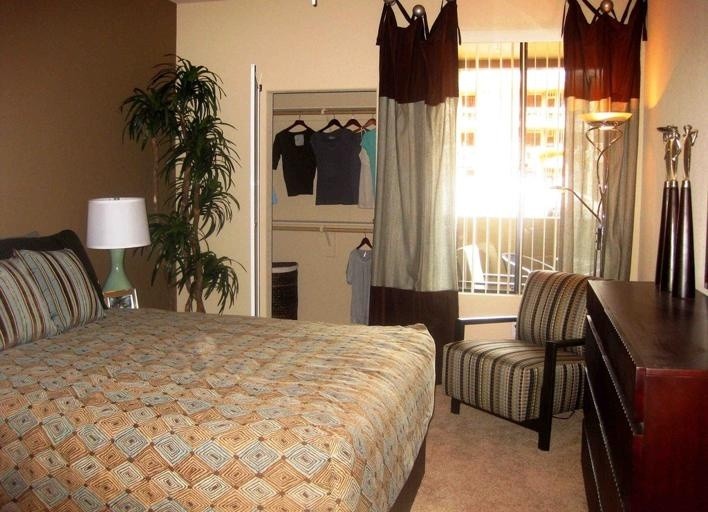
top-left (85, 192), bottom-right (152, 293)
top-left (544, 109), bottom-right (631, 278)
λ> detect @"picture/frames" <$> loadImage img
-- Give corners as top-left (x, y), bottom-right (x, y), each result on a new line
top-left (101, 289), bottom-right (140, 312)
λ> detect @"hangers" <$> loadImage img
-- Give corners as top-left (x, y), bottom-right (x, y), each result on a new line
top-left (271, 108), bottom-right (376, 139)
top-left (350, 229), bottom-right (373, 254)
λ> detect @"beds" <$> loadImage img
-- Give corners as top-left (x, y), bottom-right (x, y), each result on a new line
top-left (0, 227), bottom-right (439, 512)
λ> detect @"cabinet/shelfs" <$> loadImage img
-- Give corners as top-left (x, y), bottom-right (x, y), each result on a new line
top-left (577, 274), bottom-right (707, 512)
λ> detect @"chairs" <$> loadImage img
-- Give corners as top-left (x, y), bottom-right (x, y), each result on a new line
top-left (499, 249), bottom-right (556, 294)
top-left (456, 246), bottom-right (511, 291)
top-left (441, 269), bottom-right (599, 452)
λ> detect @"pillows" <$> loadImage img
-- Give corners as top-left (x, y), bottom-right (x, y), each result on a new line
top-left (0, 254), bottom-right (59, 353)
top-left (11, 246), bottom-right (106, 335)
